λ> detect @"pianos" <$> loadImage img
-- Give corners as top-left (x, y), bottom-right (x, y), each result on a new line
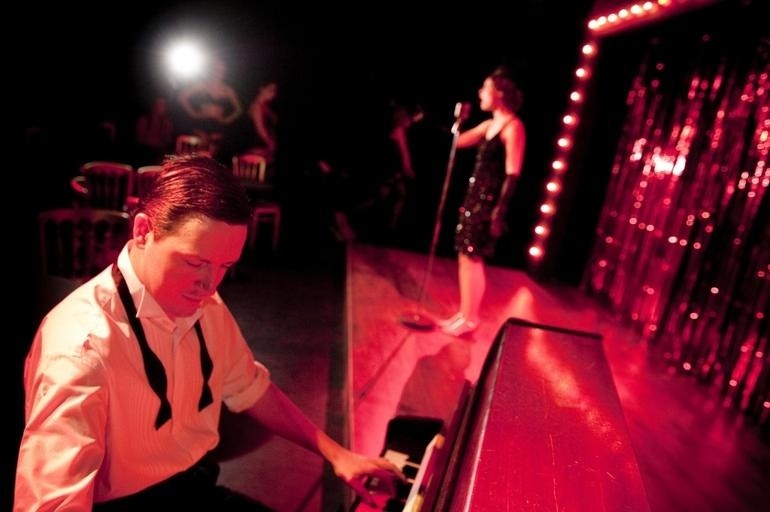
top-left (349, 317), bottom-right (649, 512)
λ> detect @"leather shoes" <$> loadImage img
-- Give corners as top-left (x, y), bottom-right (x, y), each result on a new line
top-left (435, 312), bottom-right (479, 338)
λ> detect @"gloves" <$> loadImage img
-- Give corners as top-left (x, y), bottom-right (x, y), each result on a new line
top-left (490, 175), bottom-right (521, 237)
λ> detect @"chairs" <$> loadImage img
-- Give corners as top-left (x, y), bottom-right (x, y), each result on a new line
top-left (39, 134), bottom-right (283, 279)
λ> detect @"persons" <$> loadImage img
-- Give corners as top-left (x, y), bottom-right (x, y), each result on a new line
top-left (437, 67), bottom-right (528, 336)
top-left (360, 100), bottom-right (427, 228)
top-left (177, 56), bottom-right (243, 143)
top-left (133, 92), bottom-right (176, 150)
top-left (250, 74), bottom-right (284, 151)
top-left (8, 150), bottom-right (412, 512)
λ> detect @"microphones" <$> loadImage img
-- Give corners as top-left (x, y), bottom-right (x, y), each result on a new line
top-left (454, 102), bottom-right (472, 120)
